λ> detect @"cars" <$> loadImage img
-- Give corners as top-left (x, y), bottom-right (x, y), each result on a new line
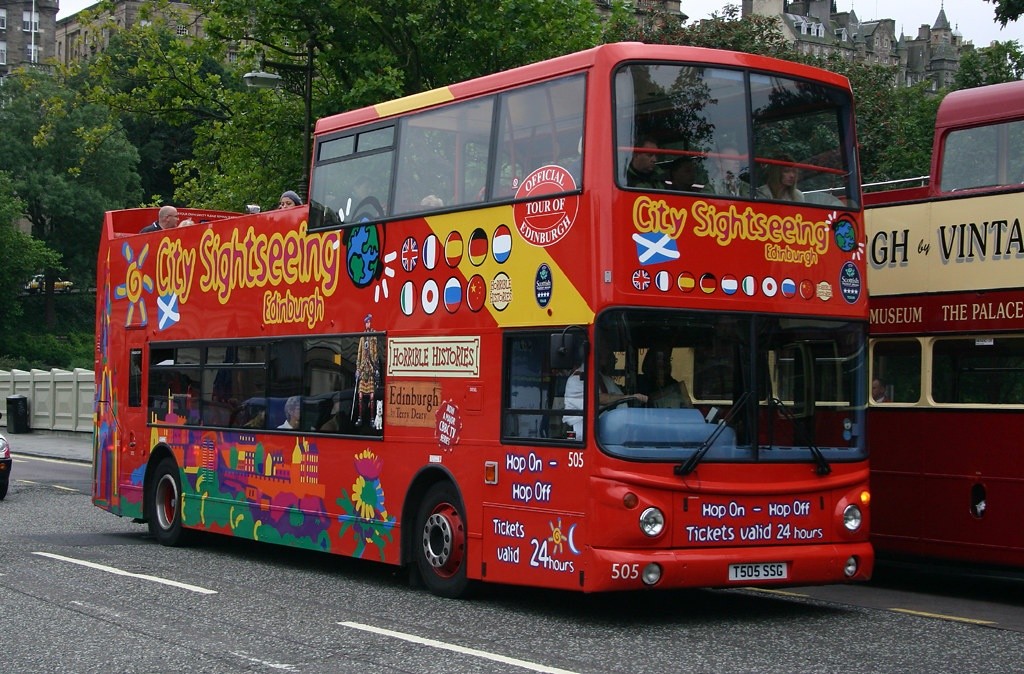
top-left (0, 412), bottom-right (13, 500)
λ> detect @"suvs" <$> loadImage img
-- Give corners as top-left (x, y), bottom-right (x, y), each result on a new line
top-left (25, 275), bottom-right (73, 295)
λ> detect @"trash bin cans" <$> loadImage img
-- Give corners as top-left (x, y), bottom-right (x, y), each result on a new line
top-left (6, 394), bottom-right (28, 434)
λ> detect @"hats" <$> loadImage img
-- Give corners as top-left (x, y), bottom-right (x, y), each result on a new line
top-left (280, 191), bottom-right (303, 206)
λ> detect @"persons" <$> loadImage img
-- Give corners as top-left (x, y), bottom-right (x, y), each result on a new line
top-left (324, 175), bottom-right (387, 227)
top-left (278, 191), bottom-right (303, 210)
top-left (277, 396), bottom-right (316, 431)
top-left (564, 136), bottom-right (583, 190)
top-left (140, 205), bottom-right (209, 234)
top-left (638, 348), bottom-right (694, 408)
top-left (563, 344), bottom-right (648, 440)
top-left (626, 136), bottom-right (669, 190)
top-left (756, 154), bottom-right (805, 204)
top-left (668, 129), bottom-right (755, 199)
top-left (241, 404), bottom-right (267, 430)
top-left (872, 377), bottom-right (892, 402)
top-left (420, 169), bottom-right (456, 208)
top-left (320, 393), bottom-right (352, 433)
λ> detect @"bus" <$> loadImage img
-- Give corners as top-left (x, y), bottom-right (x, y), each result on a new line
top-left (613, 81), bottom-right (1024, 581)
top-left (92, 41), bottom-right (873, 597)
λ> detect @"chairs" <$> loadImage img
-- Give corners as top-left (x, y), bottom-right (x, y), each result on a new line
top-left (204, 399), bottom-right (333, 432)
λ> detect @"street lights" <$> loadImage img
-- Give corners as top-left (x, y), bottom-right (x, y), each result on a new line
top-left (242, 33), bottom-right (315, 204)
